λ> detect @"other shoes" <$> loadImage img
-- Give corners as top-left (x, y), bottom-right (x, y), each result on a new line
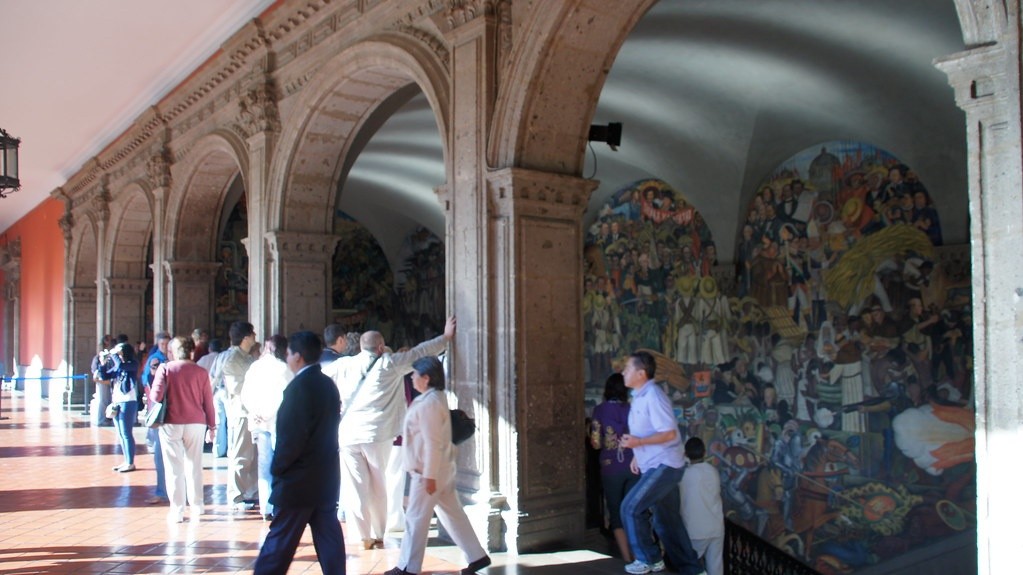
top-left (359, 540), bottom-right (371, 549)
top-left (263, 514), bottom-right (274, 520)
top-left (242, 499), bottom-right (255, 510)
top-left (167, 509), bottom-right (183, 523)
top-left (385, 522), bottom-right (404, 532)
top-left (189, 502), bottom-right (205, 515)
top-left (146, 439), bottom-right (156, 453)
top-left (145, 495), bottom-right (170, 503)
top-left (384, 567), bottom-right (417, 575)
top-left (119, 464), bottom-right (136, 472)
top-left (461, 555), bottom-right (491, 575)
top-left (113, 464), bottom-right (126, 471)
top-left (337, 509), bottom-right (346, 522)
top-left (624, 560), bottom-right (665, 574)
top-left (370, 537), bottom-right (384, 549)
top-left (698, 571), bottom-right (708, 575)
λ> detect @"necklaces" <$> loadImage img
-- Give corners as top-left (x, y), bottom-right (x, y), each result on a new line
top-left (616, 443), bottom-right (624, 463)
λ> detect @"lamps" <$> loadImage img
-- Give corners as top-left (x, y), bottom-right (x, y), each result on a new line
top-left (0, 128), bottom-right (22, 198)
top-left (587, 122), bottom-right (622, 150)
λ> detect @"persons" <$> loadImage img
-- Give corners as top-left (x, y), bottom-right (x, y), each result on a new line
top-left (91, 332), bottom-right (216, 524)
top-left (318, 316), bottom-right (491, 575)
top-left (616, 350), bottom-right (725, 575)
top-left (191, 321), bottom-right (346, 575)
top-left (589, 372), bottom-right (644, 564)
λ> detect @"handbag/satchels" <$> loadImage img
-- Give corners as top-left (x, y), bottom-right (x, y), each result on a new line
top-left (155, 362), bottom-right (167, 424)
top-left (145, 401), bottom-right (162, 428)
top-left (104, 402), bottom-right (120, 419)
top-left (450, 409), bottom-right (475, 444)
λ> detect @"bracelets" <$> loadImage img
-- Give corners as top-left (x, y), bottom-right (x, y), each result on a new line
top-left (207, 425), bottom-right (216, 431)
